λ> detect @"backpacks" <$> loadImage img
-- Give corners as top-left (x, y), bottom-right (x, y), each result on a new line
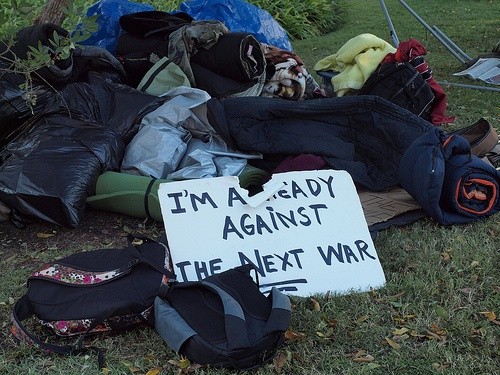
top-left (150, 262), bottom-right (294, 370)
top-left (355, 61), bottom-right (436, 117)
top-left (9, 232), bottom-right (173, 365)
